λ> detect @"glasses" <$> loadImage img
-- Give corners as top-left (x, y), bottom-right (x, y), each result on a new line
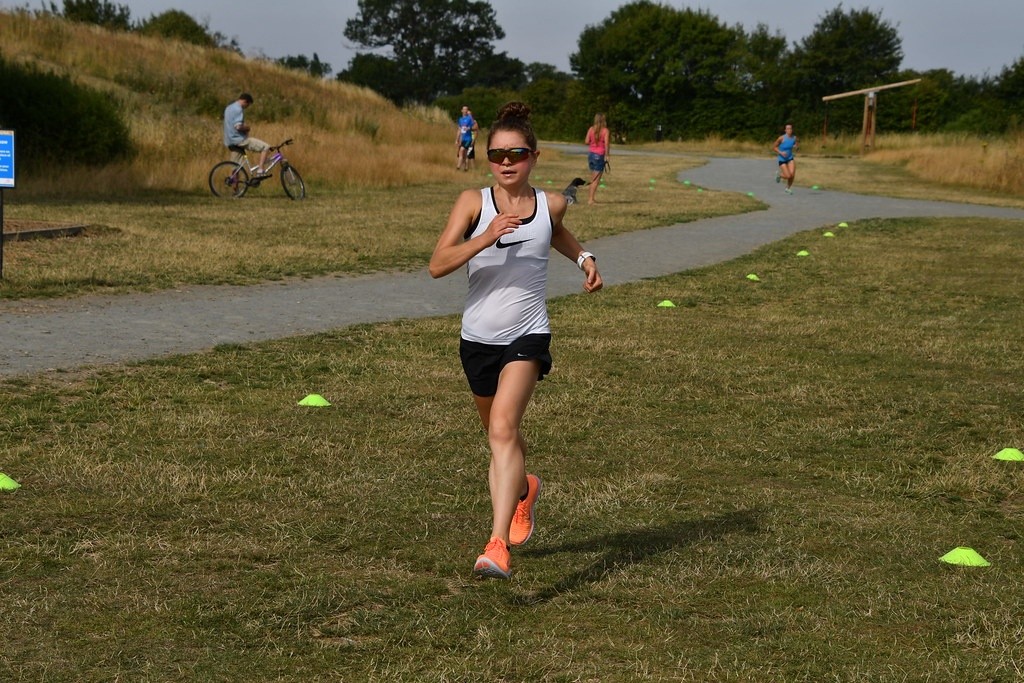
top-left (486, 148), bottom-right (532, 163)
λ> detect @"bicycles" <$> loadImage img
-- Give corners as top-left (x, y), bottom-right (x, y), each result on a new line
top-left (208, 136), bottom-right (305, 201)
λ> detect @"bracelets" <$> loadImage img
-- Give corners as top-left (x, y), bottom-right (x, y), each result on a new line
top-left (576, 251), bottom-right (597, 269)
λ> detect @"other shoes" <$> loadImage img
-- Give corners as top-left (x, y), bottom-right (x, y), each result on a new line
top-left (257, 170), bottom-right (273, 178)
top-left (776, 171), bottom-right (781, 182)
top-left (784, 188), bottom-right (792, 194)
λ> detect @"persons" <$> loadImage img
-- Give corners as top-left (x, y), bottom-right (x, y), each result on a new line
top-left (774, 123), bottom-right (798, 195)
top-left (430, 103), bottom-right (604, 578)
top-left (585, 113), bottom-right (609, 204)
top-left (223, 94), bottom-right (272, 196)
top-left (455, 105), bottom-right (478, 171)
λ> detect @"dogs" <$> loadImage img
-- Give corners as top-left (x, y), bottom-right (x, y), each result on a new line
top-left (562, 177), bottom-right (586, 205)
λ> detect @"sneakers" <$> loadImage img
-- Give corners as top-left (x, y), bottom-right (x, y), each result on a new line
top-left (509, 474), bottom-right (540, 546)
top-left (473, 536), bottom-right (512, 577)
